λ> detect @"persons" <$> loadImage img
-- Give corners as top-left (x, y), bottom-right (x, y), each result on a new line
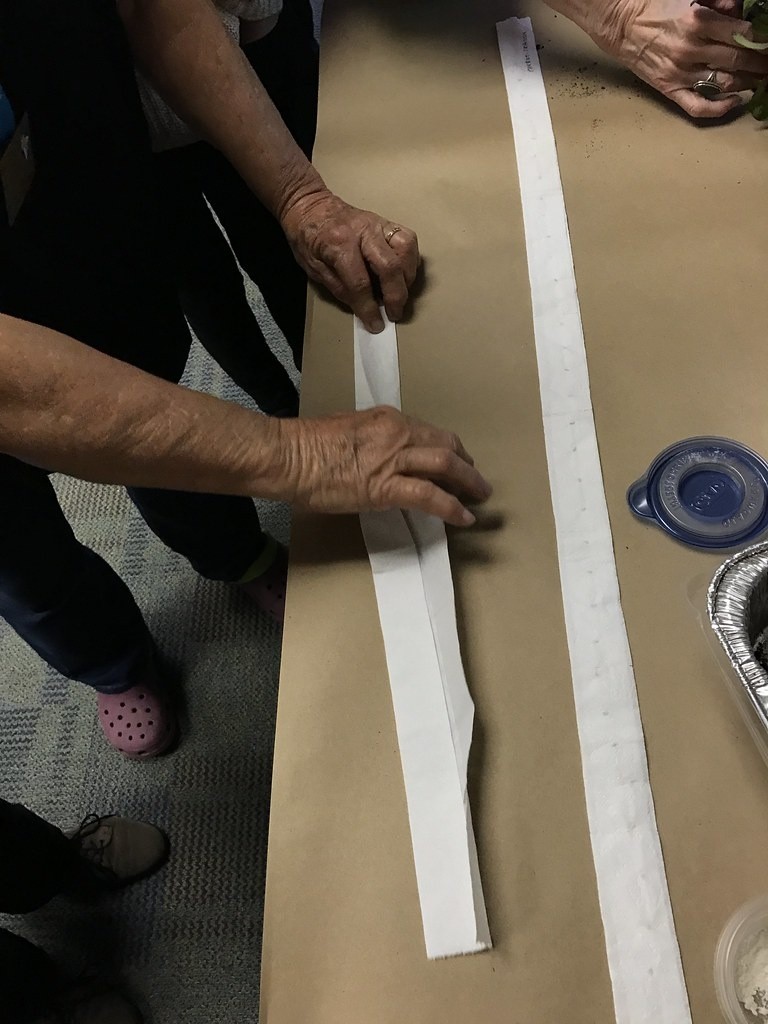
top-left (134, 0), bottom-right (768, 372)
top-left (0, 0), bottom-right (493, 760)
top-left (0, 798), bottom-right (169, 1024)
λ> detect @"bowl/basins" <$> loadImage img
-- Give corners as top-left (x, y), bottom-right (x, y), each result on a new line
top-left (712, 897), bottom-right (767, 1023)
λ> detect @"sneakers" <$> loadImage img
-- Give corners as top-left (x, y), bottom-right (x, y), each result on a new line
top-left (62, 814), bottom-right (170, 892)
top-left (32, 976), bottom-right (143, 1024)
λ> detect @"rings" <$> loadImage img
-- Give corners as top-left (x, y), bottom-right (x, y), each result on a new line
top-left (385, 227), bottom-right (401, 243)
top-left (692, 70), bottom-right (722, 95)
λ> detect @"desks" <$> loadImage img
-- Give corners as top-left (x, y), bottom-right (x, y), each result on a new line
top-left (259, 1), bottom-right (768, 1024)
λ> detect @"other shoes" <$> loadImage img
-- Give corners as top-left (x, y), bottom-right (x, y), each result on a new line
top-left (266, 395), bottom-right (300, 418)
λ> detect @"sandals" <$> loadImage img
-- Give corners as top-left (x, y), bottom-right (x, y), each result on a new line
top-left (228, 533), bottom-right (289, 629)
top-left (99, 679), bottom-right (175, 759)
